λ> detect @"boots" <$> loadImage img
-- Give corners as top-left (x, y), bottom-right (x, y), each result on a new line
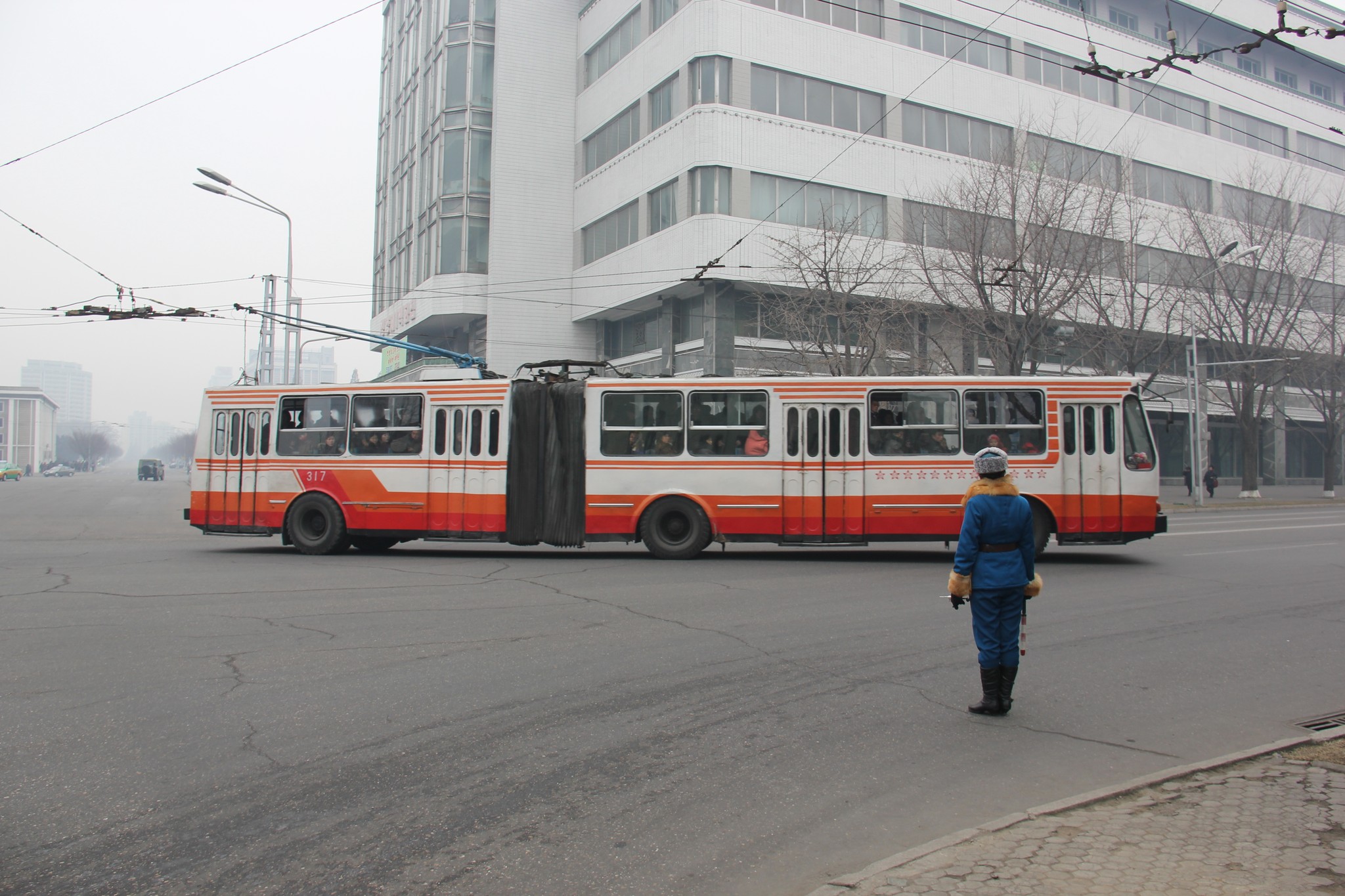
top-left (968, 665), bottom-right (1000, 716)
top-left (998, 664), bottom-right (1018, 715)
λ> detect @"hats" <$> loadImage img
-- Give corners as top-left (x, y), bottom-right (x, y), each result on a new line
top-left (987, 434), bottom-right (1000, 444)
top-left (973, 446), bottom-right (1008, 474)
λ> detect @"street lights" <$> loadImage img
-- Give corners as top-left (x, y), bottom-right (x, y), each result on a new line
top-left (1191, 239), bottom-right (1260, 504)
top-left (193, 166), bottom-right (291, 385)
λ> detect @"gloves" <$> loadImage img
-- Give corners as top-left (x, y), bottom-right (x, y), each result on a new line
top-left (950, 592), bottom-right (965, 610)
top-left (1022, 593), bottom-right (1032, 602)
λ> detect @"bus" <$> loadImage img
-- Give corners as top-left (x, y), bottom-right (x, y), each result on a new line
top-left (184, 373), bottom-right (1168, 560)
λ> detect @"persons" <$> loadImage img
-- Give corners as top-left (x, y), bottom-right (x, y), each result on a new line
top-left (187, 463), bottom-right (191, 475)
top-left (1202, 466), bottom-right (1219, 498)
top-left (38, 460), bottom-right (94, 474)
top-left (230, 413), bottom-right (271, 456)
top-left (870, 397), bottom-right (1039, 457)
top-left (23, 464), bottom-right (33, 477)
top-left (601, 395), bottom-right (767, 456)
top-left (436, 409), bottom-right (499, 455)
top-left (947, 447), bottom-right (1043, 716)
top-left (1183, 467), bottom-right (1192, 497)
top-left (152, 463), bottom-right (159, 481)
top-left (276, 395), bottom-right (423, 456)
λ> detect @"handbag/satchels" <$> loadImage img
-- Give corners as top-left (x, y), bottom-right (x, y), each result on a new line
top-left (1213, 480), bottom-right (1218, 488)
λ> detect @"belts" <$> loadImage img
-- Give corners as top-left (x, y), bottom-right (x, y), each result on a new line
top-left (978, 540), bottom-right (1018, 553)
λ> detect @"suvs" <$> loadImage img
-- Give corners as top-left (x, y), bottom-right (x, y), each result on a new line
top-left (138, 458), bottom-right (165, 481)
